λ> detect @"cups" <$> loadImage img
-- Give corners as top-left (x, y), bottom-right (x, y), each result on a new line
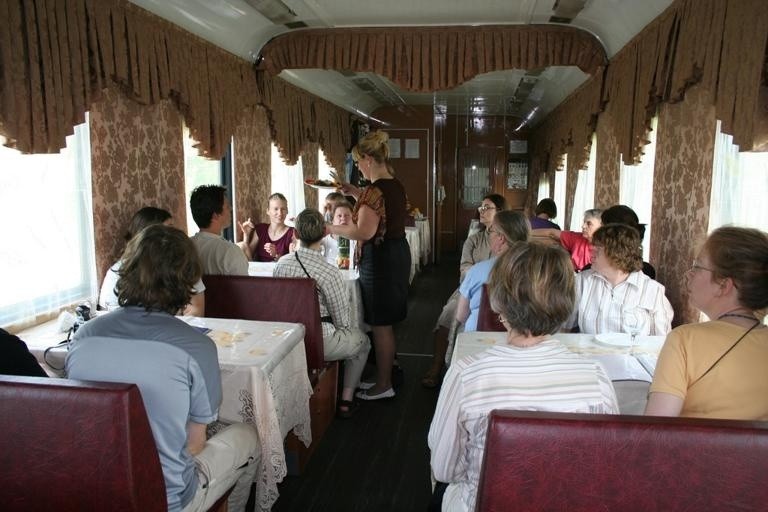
top-left (318, 245), bottom-right (339, 271)
top-left (105, 298), bottom-right (121, 313)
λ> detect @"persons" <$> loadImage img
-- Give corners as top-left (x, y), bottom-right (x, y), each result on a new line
top-left (190, 186), bottom-right (249, 276)
top-left (456, 195), bottom-right (529, 331)
top-left (328, 192), bottom-right (357, 258)
top-left (271, 210), bottom-right (371, 415)
top-left (531, 198), bottom-right (560, 230)
top-left (564, 224), bottom-right (674, 335)
top-left (642, 228), bottom-right (768, 421)
top-left (427, 244), bottom-right (621, 512)
top-left (559, 205), bottom-right (656, 279)
top-left (65, 224), bottom-right (262, 512)
top-left (99, 206), bottom-right (206, 317)
top-left (325, 128), bottom-right (411, 400)
top-left (236, 192), bottom-right (296, 261)
top-left (0, 328), bottom-right (49, 377)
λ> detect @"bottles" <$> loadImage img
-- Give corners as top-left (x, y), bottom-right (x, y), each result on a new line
top-left (73, 306), bottom-right (90, 336)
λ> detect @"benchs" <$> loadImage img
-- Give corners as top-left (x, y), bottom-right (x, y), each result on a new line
top-left (202, 270), bottom-right (341, 452)
top-left (474, 282), bottom-right (582, 333)
top-left (0, 366), bottom-right (235, 509)
top-left (470, 410), bottom-right (767, 512)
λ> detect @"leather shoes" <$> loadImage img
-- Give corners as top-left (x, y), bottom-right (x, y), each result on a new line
top-left (339, 382), bottom-right (395, 418)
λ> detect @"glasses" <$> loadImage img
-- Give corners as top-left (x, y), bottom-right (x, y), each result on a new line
top-left (478, 207), bottom-right (498, 212)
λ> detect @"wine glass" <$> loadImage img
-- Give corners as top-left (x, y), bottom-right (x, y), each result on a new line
top-left (620, 304), bottom-right (648, 358)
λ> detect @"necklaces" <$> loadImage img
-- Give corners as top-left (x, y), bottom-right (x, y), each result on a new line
top-left (718, 314), bottom-right (759, 321)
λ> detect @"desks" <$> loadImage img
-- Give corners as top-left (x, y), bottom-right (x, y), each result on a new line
top-left (404, 217), bottom-right (429, 286)
top-left (241, 258), bottom-right (365, 364)
top-left (456, 327), bottom-right (676, 418)
top-left (22, 306), bottom-right (306, 501)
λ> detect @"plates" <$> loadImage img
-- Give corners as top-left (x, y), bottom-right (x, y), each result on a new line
top-left (596, 330), bottom-right (639, 348)
top-left (305, 178), bottom-right (341, 189)
